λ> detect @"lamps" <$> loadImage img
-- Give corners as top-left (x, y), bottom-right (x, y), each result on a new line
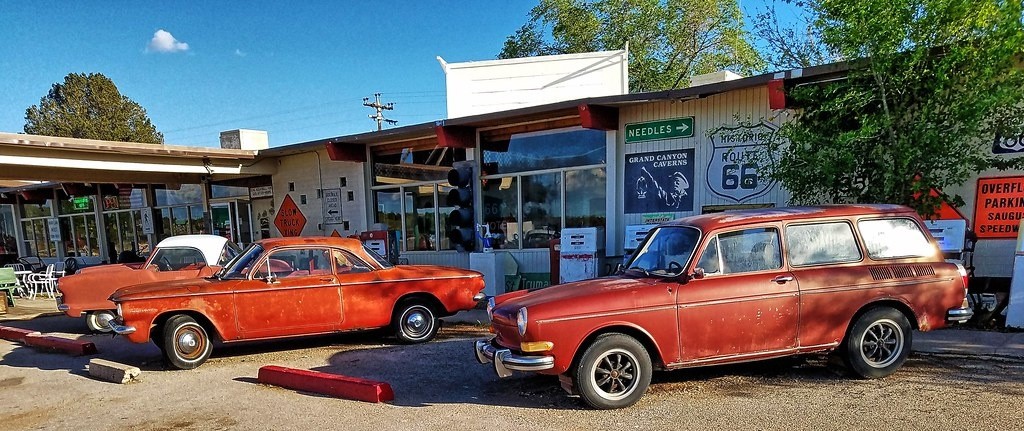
top-left (773, 109), bottom-right (790, 117)
top-left (68, 194), bottom-right (74, 202)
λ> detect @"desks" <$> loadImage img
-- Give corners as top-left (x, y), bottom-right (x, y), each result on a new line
top-left (10, 270), bottom-right (32, 298)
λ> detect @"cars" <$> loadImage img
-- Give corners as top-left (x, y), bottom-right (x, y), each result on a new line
top-left (55, 235), bottom-right (294, 333)
top-left (474, 204), bottom-right (974, 409)
top-left (107, 236), bottom-right (487, 370)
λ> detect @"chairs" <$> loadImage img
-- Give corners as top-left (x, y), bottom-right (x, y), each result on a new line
top-left (748, 242), bottom-right (775, 270)
top-left (0, 258), bottom-right (77, 307)
top-left (699, 241), bottom-right (729, 273)
